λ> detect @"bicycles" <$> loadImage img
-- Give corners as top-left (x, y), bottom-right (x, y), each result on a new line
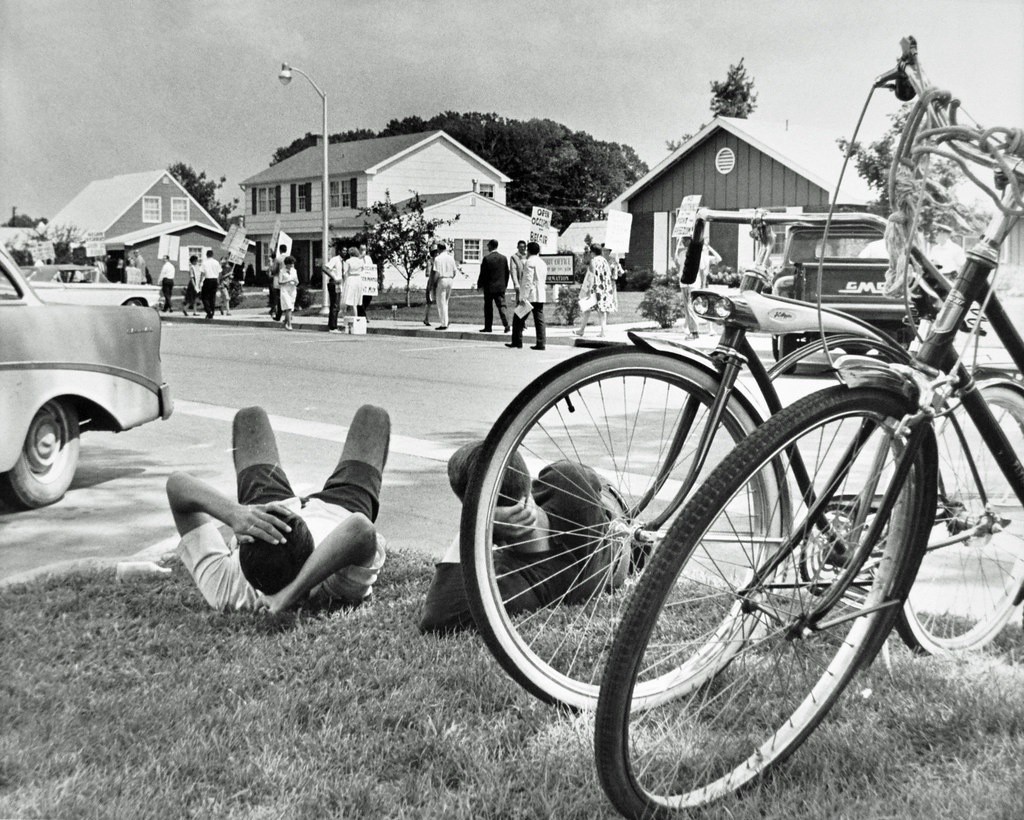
top-left (460, 207), bottom-right (1024, 718)
top-left (593, 35), bottom-right (1024, 819)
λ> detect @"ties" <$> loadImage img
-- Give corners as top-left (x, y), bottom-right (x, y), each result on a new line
top-left (342, 259), bottom-right (344, 281)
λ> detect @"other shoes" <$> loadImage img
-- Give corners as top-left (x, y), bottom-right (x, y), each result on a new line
top-left (273, 317), bottom-right (280, 321)
top-left (504, 326), bottom-right (510, 333)
top-left (329, 328), bottom-right (342, 334)
top-left (183, 309), bottom-right (189, 315)
top-left (505, 342), bottom-right (523, 348)
top-left (284, 318), bottom-right (293, 331)
top-left (480, 328), bottom-right (492, 332)
top-left (597, 334), bottom-right (606, 337)
top-left (193, 313), bottom-right (199, 315)
top-left (530, 346), bottom-right (545, 350)
top-left (423, 320), bottom-right (432, 326)
top-left (435, 325), bottom-right (446, 330)
top-left (572, 330), bottom-right (584, 336)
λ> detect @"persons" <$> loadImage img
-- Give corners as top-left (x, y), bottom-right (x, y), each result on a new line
top-left (672, 236), bottom-right (723, 339)
top-left (114, 251), bottom-right (175, 312)
top-left (181, 250), bottom-right (234, 319)
top-left (321, 244), bottom-right (374, 334)
top-left (422, 242), bottom-right (457, 330)
top-left (510, 240), bottom-right (527, 330)
top-left (166, 404), bottom-right (391, 614)
top-left (477, 239), bottom-right (510, 333)
top-left (504, 242), bottom-right (547, 350)
top-left (268, 245), bottom-right (299, 330)
top-left (572, 244), bottom-right (619, 336)
top-left (420, 440), bottom-right (631, 638)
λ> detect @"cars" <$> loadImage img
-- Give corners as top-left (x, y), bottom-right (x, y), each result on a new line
top-left (20, 264), bottom-right (165, 310)
top-left (0, 248), bottom-right (173, 510)
top-left (772, 212), bottom-right (921, 375)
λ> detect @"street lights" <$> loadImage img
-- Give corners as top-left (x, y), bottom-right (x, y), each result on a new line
top-left (278, 62), bottom-right (330, 316)
